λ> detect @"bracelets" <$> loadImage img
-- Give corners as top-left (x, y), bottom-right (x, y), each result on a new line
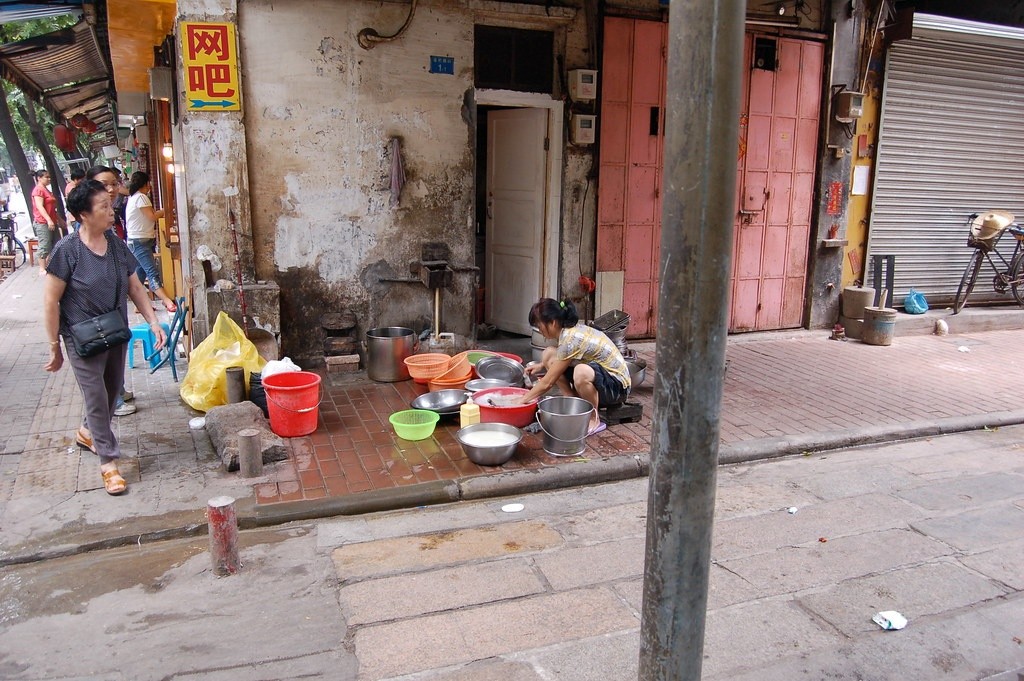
top-left (49, 340), bottom-right (61, 344)
top-left (149, 321), bottom-right (159, 326)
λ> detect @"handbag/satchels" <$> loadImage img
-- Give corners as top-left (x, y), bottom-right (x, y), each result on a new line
top-left (69, 309), bottom-right (132, 358)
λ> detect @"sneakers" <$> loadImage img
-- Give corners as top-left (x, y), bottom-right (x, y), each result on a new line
top-left (122, 391), bottom-right (133, 401)
top-left (113, 402), bottom-right (137, 416)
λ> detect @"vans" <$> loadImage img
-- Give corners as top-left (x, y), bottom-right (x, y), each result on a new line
top-left (0, 168), bottom-right (11, 211)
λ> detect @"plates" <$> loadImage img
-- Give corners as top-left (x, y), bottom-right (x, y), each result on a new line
top-left (464, 378), bottom-right (510, 392)
top-left (475, 356), bottom-right (525, 385)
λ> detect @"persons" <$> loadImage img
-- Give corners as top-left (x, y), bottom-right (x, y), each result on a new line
top-left (43, 180), bottom-right (168, 497)
top-left (32, 170), bottom-right (68, 275)
top-left (64, 165), bottom-right (131, 240)
top-left (124, 172), bottom-right (179, 313)
top-left (11, 173), bottom-right (20, 193)
top-left (512, 296), bottom-right (632, 433)
top-left (76, 166), bottom-right (138, 414)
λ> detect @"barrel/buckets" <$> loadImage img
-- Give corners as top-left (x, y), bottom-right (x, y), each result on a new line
top-left (261, 371), bottom-right (323, 438)
top-left (536, 397), bottom-right (598, 458)
top-left (361, 327), bottom-right (419, 383)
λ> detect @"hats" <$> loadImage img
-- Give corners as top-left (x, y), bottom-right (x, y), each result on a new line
top-left (971, 209), bottom-right (1014, 239)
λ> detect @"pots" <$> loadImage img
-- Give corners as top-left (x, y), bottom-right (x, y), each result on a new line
top-left (528, 327), bottom-right (559, 361)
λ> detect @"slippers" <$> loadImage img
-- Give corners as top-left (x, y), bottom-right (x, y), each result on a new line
top-left (588, 422), bottom-right (606, 435)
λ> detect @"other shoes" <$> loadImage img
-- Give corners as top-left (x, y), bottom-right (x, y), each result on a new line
top-left (162, 301), bottom-right (177, 312)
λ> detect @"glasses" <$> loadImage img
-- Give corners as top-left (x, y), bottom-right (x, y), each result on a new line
top-left (103, 182), bottom-right (120, 190)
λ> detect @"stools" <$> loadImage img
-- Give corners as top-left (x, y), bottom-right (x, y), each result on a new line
top-left (128, 277), bottom-right (157, 301)
top-left (129, 323), bottom-right (175, 369)
top-left (28, 240), bottom-right (39, 267)
top-left (0, 256), bottom-right (15, 278)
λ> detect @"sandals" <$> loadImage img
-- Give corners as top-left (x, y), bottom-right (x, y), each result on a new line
top-left (101, 470), bottom-right (127, 495)
top-left (76, 431), bottom-right (98, 455)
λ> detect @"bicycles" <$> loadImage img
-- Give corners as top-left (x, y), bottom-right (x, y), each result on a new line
top-left (0, 211), bottom-right (26, 268)
top-left (953, 213), bottom-right (1024, 314)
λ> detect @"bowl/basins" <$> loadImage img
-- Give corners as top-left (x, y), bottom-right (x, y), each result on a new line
top-left (410, 389), bottom-right (469, 421)
top-left (456, 422), bottom-right (524, 467)
top-left (469, 387), bottom-right (539, 427)
top-left (605, 326), bottom-right (648, 389)
top-left (427, 373), bottom-right (472, 391)
top-left (498, 352), bottom-right (522, 364)
top-left (466, 348), bottom-right (501, 378)
